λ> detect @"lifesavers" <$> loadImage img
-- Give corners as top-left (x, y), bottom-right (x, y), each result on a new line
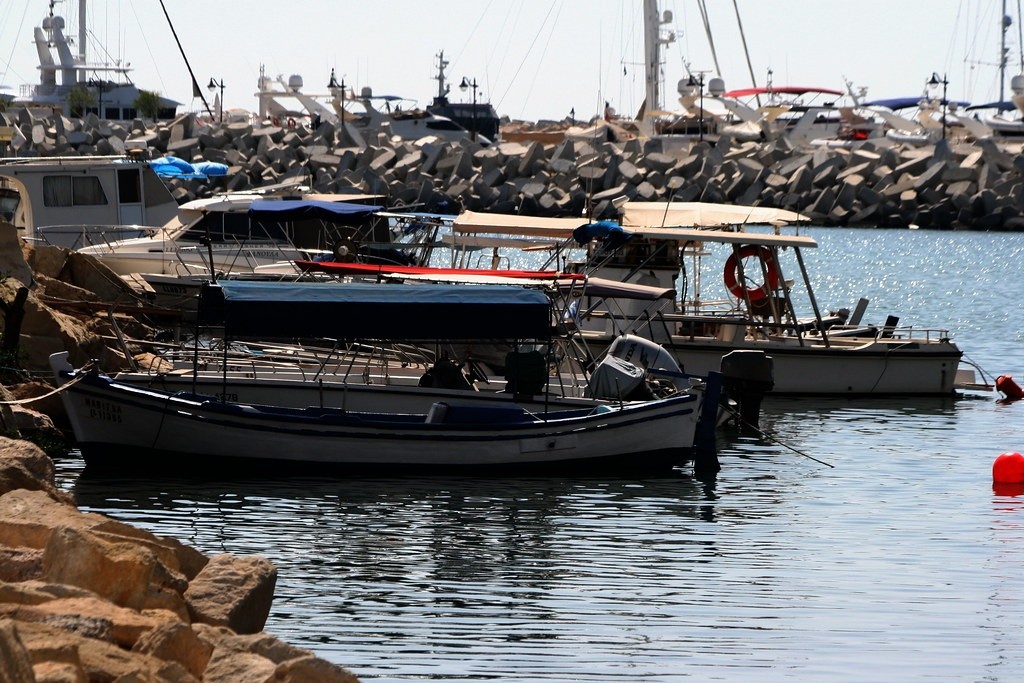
top-left (723, 244), bottom-right (779, 302)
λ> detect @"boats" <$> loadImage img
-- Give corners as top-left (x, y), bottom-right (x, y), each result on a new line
top-left (0, 0), bottom-right (996, 403)
top-left (47, 281), bottom-right (709, 484)
